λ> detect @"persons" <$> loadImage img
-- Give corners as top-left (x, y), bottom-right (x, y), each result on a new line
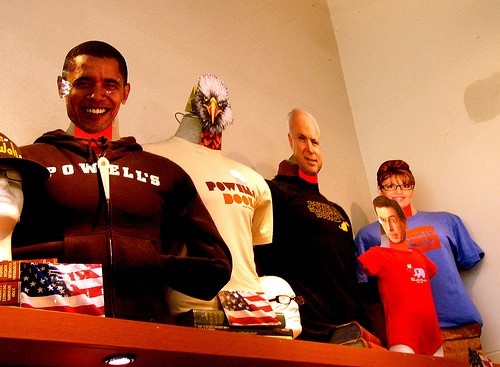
top-left (358, 159), bottom-right (485, 330)
top-left (134, 113), bottom-right (274, 313)
top-left (0, 132), bottom-right (51, 262)
top-left (376, 160), bottom-right (415, 208)
top-left (57, 42), bottom-right (132, 132)
top-left (10, 41), bottom-right (233, 326)
top-left (357, 195), bottom-right (446, 358)
top-left (373, 196), bottom-right (408, 244)
top-left (258, 275), bottom-right (303, 340)
top-left (287, 108), bottom-right (323, 175)
top-left (253, 107), bottom-right (370, 341)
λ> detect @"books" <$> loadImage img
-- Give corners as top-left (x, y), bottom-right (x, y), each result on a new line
top-left (439, 327), bottom-right (482, 355)
top-left (165, 305), bottom-right (294, 339)
top-left (319, 320), bottom-right (390, 352)
top-left (0, 258), bottom-right (58, 307)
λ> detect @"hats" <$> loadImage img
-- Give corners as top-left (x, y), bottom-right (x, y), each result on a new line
top-left (0, 131), bottom-right (50, 189)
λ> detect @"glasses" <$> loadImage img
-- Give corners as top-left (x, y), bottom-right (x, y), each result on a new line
top-left (0, 167), bottom-right (26, 182)
top-left (268, 294), bottom-right (305, 305)
top-left (381, 182), bottom-right (414, 191)
